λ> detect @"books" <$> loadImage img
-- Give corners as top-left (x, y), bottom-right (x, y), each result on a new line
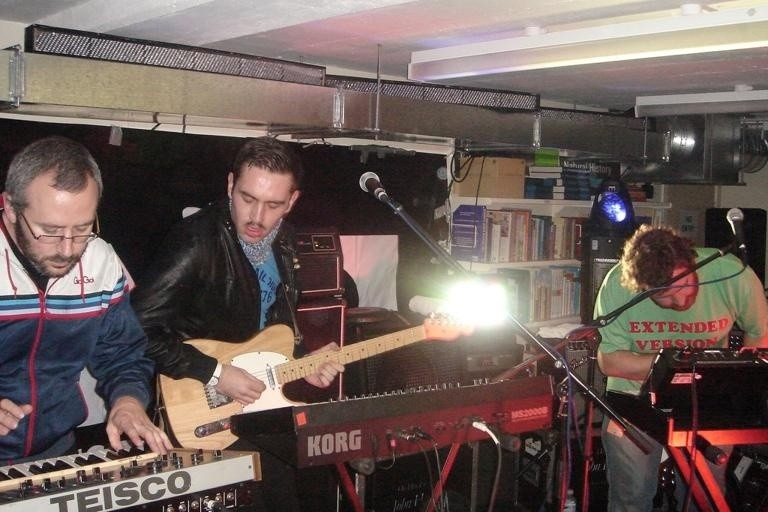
top-left (445, 152), bottom-right (623, 330)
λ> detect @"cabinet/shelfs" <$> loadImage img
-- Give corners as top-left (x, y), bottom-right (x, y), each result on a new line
top-left (447, 197), bottom-right (673, 329)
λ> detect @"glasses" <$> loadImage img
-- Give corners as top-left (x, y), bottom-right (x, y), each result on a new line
top-left (16, 204), bottom-right (102, 246)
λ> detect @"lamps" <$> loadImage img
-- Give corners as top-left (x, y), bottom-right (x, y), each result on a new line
top-left (407, 5), bottom-right (768, 83)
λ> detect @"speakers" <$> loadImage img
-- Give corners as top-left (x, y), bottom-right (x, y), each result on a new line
top-left (289, 226), bottom-right (346, 300)
top-left (355, 311), bottom-right (469, 396)
top-left (282, 300), bottom-right (346, 404)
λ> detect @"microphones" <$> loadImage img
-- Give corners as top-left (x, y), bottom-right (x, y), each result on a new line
top-left (726, 208), bottom-right (749, 266)
top-left (358, 171), bottom-right (388, 202)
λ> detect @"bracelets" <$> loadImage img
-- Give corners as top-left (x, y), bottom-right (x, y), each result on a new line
top-left (206, 362), bottom-right (223, 387)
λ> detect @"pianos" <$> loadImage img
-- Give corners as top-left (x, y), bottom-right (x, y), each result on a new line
top-left (0, 444), bottom-right (264, 512)
top-left (230, 373), bottom-right (560, 471)
top-left (607, 347), bottom-right (768, 449)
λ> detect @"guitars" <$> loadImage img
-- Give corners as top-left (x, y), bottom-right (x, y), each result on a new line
top-left (160, 312), bottom-right (473, 451)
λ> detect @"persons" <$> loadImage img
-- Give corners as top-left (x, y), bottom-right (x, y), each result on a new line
top-left (0, 133), bottom-right (174, 467)
top-left (128, 134), bottom-right (348, 443)
top-left (588, 222), bottom-right (768, 511)
